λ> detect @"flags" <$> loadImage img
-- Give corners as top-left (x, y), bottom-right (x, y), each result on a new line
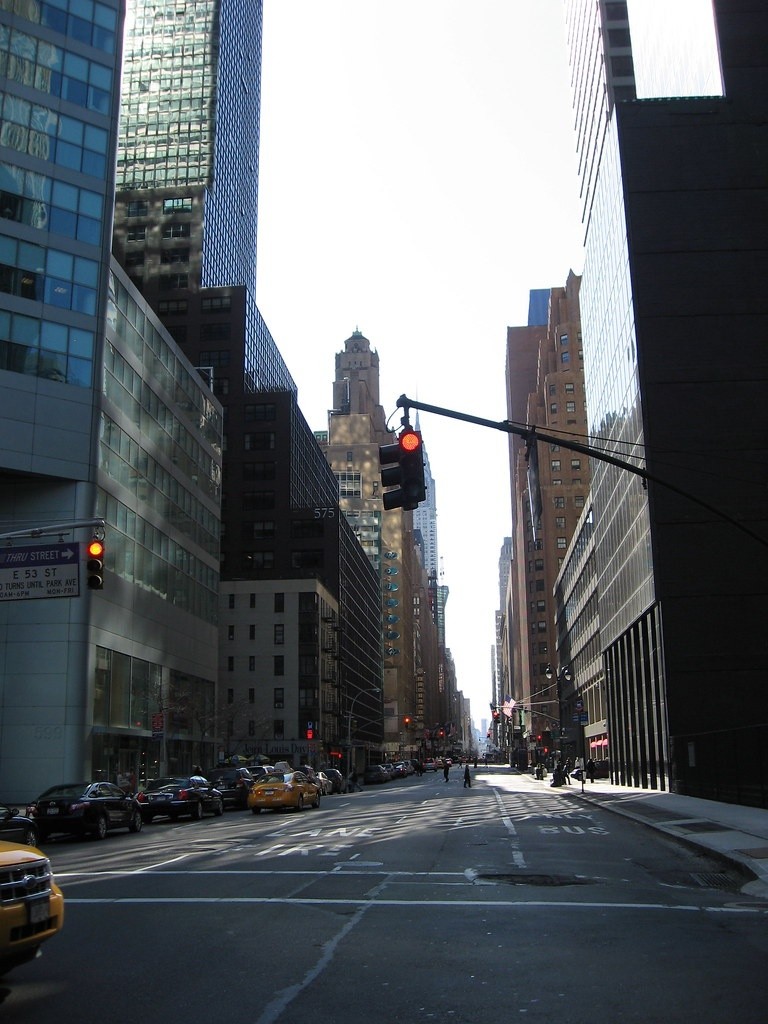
top-left (501, 695), bottom-right (516, 718)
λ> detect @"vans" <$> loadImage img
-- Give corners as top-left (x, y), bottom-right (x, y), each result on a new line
top-left (246, 765), bottom-right (274, 783)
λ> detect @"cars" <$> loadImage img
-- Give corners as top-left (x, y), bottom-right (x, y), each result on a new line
top-left (138, 774), bottom-right (224, 825)
top-left (364, 757), bottom-right (452, 785)
top-left (316, 769), bottom-right (348, 795)
top-left (204, 767), bottom-right (254, 810)
top-left (25, 781), bottom-right (143, 842)
top-left (0, 840), bottom-right (64, 970)
top-left (247, 770), bottom-right (321, 814)
top-left (0, 804), bottom-right (39, 848)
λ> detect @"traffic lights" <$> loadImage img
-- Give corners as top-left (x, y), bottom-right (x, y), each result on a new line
top-left (406, 717), bottom-right (410, 728)
top-left (86, 540), bottom-right (105, 591)
top-left (494, 713), bottom-right (501, 723)
top-left (306, 719), bottom-right (314, 739)
top-left (440, 728), bottom-right (443, 736)
top-left (399, 430), bottom-right (427, 502)
top-left (379, 443), bottom-right (401, 511)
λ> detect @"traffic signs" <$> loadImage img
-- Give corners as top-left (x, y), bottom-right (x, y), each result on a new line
top-left (0, 541), bottom-right (81, 601)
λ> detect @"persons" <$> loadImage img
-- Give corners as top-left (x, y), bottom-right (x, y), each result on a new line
top-left (464, 765), bottom-right (471, 788)
top-left (551, 758), bottom-right (571, 787)
top-left (457, 759), bottom-right (463, 769)
top-left (586, 758), bottom-right (595, 783)
top-left (473, 757), bottom-right (478, 768)
top-left (442, 762), bottom-right (449, 783)
top-left (412, 760), bottom-right (422, 777)
top-left (349, 768), bottom-right (363, 793)
top-left (484, 759), bottom-right (488, 768)
top-left (194, 766), bottom-right (202, 776)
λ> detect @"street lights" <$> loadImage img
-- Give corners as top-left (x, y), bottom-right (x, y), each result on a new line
top-left (347, 687), bottom-right (381, 771)
top-left (546, 662), bottom-right (572, 766)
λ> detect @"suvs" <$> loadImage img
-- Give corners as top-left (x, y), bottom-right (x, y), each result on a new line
top-left (291, 765), bottom-right (322, 796)
